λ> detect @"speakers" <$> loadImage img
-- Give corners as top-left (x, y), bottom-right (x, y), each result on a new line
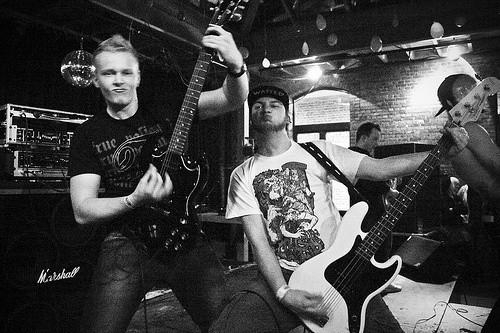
top-left (394, 234), bottom-right (457, 285)
top-left (0, 187), bottom-right (109, 333)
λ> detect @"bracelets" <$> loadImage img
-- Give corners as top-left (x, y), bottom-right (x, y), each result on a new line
top-left (275, 285), bottom-right (291, 303)
top-left (124, 196), bottom-right (139, 210)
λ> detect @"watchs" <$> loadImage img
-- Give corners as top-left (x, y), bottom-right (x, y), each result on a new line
top-left (228, 61), bottom-right (247, 78)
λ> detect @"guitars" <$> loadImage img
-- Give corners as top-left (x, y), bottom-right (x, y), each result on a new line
top-left (135, 0), bottom-right (249, 249)
top-left (288, 76), bottom-right (499, 333)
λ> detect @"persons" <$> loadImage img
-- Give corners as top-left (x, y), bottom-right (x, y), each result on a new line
top-left (206, 85), bottom-right (469, 333)
top-left (435, 74), bottom-right (500, 250)
top-left (68, 23), bottom-right (249, 333)
top-left (348, 123), bottom-right (401, 292)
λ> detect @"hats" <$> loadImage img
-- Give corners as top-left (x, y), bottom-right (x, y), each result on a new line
top-left (248, 86), bottom-right (289, 108)
top-left (435, 74), bottom-right (477, 118)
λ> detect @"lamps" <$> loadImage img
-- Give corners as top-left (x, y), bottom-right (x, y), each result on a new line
top-left (239, 38), bottom-right (249, 59)
top-left (262, 27), bottom-right (270, 68)
top-left (327, 14), bottom-right (338, 46)
top-left (430, 21), bottom-right (444, 39)
top-left (60, 37), bottom-right (94, 88)
top-left (302, 15), bottom-right (310, 56)
top-left (370, 34), bottom-right (383, 53)
top-left (315, 13), bottom-right (326, 31)
